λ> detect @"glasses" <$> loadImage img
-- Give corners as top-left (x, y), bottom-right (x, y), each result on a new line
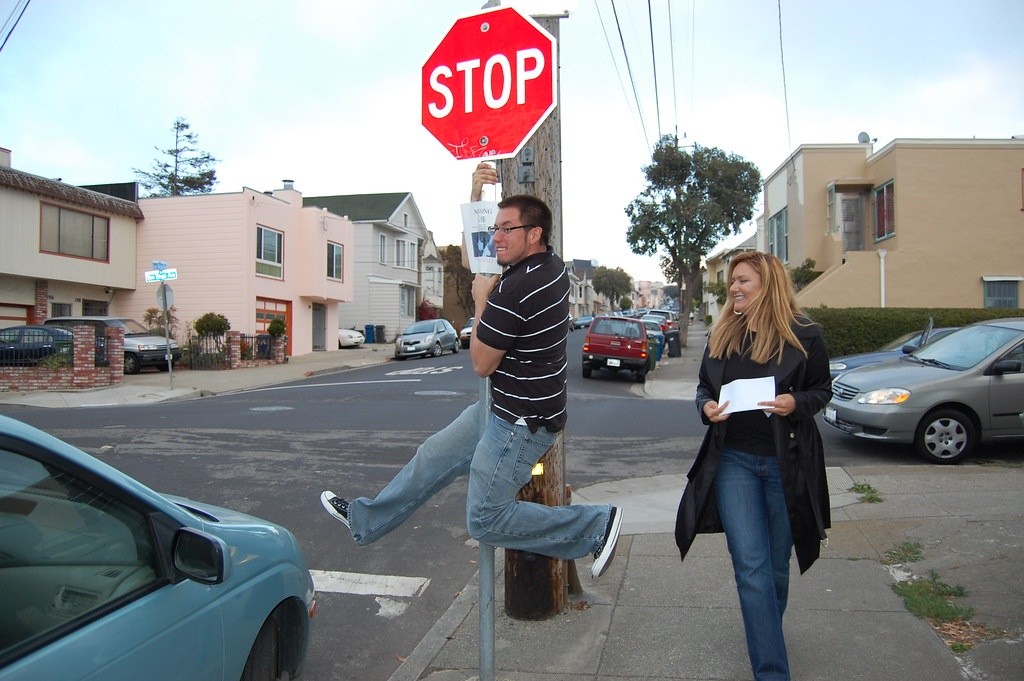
top-left (488, 224), bottom-right (537, 235)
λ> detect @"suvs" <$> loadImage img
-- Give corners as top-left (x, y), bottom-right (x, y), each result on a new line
top-left (44, 315), bottom-right (181, 375)
top-left (459, 317), bottom-right (475, 348)
top-left (581, 316), bottom-right (652, 383)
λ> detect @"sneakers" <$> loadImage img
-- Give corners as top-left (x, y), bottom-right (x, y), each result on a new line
top-left (321, 490), bottom-right (356, 540)
top-left (591, 507), bottom-right (624, 579)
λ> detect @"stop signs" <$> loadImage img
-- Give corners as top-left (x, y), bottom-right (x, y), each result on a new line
top-left (421, 5), bottom-right (556, 161)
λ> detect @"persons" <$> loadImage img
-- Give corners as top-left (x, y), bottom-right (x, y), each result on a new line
top-left (675, 251), bottom-right (834, 681)
top-left (320, 162), bottom-right (625, 579)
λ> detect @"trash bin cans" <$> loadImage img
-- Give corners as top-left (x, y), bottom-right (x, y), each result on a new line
top-left (257, 335), bottom-right (271, 358)
top-left (284, 337), bottom-right (289, 361)
top-left (365, 325), bottom-right (375, 343)
top-left (376, 325), bottom-right (386, 343)
top-left (665, 330), bottom-right (682, 357)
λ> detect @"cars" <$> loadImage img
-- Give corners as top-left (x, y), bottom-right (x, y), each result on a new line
top-left (822, 314), bottom-right (1024, 463)
top-left (596, 309), bottom-right (680, 352)
top-left (568, 313), bottom-right (575, 332)
top-left (1, 415), bottom-right (320, 681)
top-left (336, 329), bottom-right (365, 349)
top-left (574, 317), bottom-right (594, 329)
top-left (396, 320), bottom-right (461, 359)
top-left (0, 326), bottom-right (104, 367)
